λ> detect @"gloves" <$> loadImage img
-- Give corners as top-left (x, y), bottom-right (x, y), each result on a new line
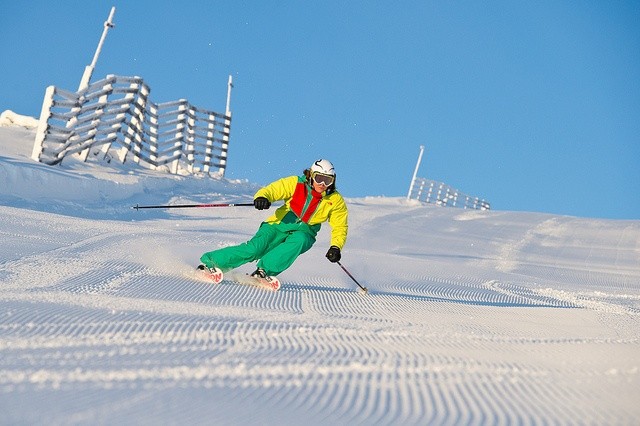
top-left (254, 196), bottom-right (270, 210)
top-left (326, 245), bottom-right (341, 262)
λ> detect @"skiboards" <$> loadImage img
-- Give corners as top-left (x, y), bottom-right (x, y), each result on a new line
top-left (173, 256), bottom-right (281, 292)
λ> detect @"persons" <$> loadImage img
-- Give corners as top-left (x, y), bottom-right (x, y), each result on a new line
top-left (198, 159), bottom-right (349, 288)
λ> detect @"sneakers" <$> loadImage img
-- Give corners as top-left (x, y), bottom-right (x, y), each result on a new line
top-left (196, 265), bottom-right (209, 272)
top-left (251, 267), bottom-right (266, 278)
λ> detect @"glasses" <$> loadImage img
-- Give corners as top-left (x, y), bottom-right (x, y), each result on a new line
top-left (312, 172), bottom-right (334, 187)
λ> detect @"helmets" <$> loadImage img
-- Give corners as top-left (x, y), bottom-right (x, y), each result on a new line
top-left (309, 158), bottom-right (336, 194)
